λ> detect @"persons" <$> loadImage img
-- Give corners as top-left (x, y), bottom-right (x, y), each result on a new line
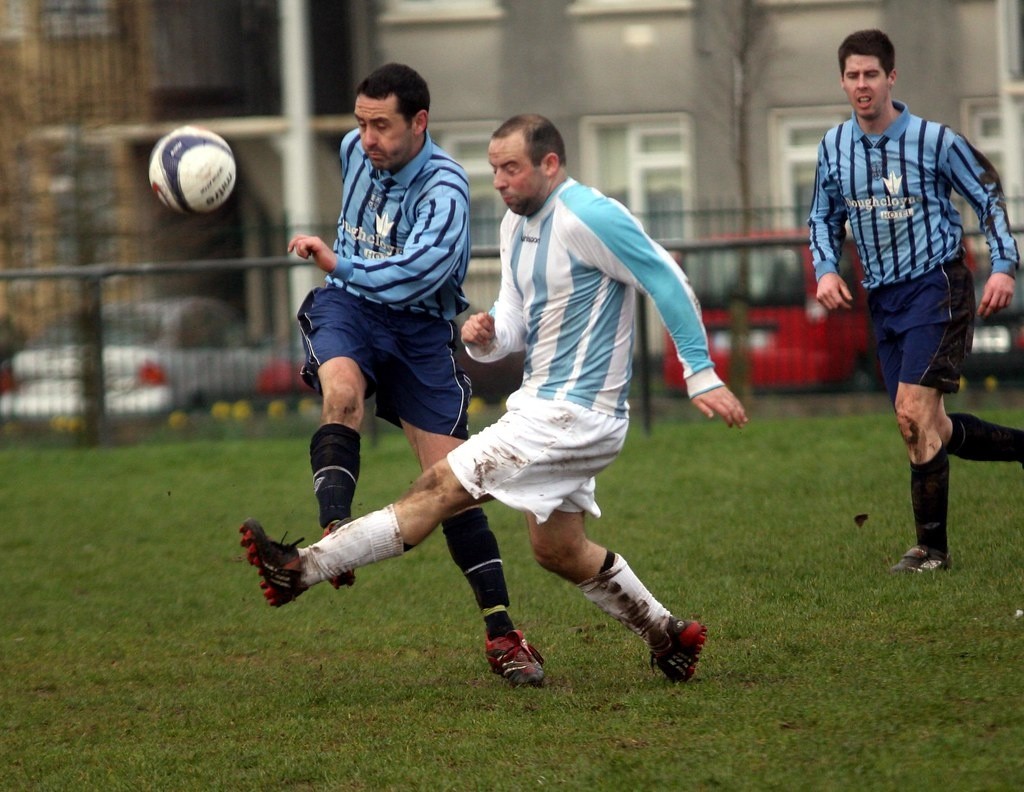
top-left (809, 30), bottom-right (1024, 574)
top-left (286, 63), bottom-right (544, 687)
top-left (243, 113), bottom-right (748, 682)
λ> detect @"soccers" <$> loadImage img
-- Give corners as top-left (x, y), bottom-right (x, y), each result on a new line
top-left (146, 125), bottom-right (239, 217)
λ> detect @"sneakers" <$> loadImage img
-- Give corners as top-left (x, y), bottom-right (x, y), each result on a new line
top-left (322, 518), bottom-right (356, 589)
top-left (890, 547), bottom-right (952, 574)
top-left (238, 517), bottom-right (309, 608)
top-left (649, 617), bottom-right (707, 685)
top-left (485, 627), bottom-right (544, 687)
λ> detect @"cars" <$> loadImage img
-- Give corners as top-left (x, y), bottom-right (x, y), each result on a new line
top-left (965, 274), bottom-right (1023, 390)
top-left (1, 296), bottom-right (275, 426)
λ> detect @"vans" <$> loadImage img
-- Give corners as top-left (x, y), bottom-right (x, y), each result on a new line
top-left (661, 228), bottom-right (878, 395)
